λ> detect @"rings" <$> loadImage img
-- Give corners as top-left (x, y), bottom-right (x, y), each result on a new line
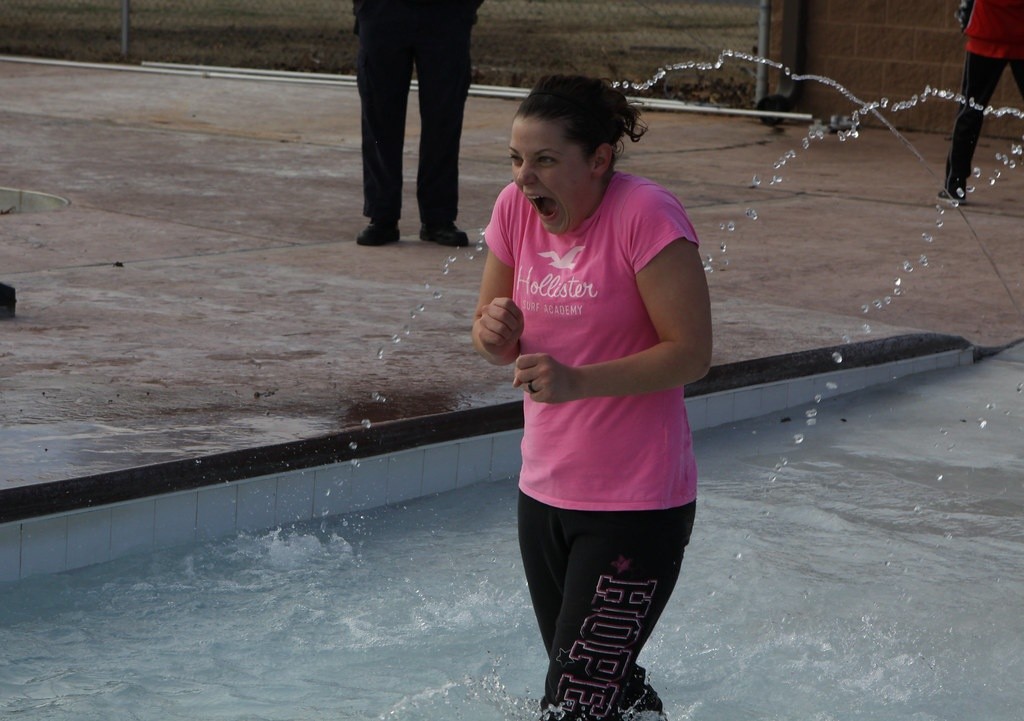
top-left (528, 381), bottom-right (537, 393)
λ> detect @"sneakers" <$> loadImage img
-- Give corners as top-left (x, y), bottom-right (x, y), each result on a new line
top-left (356, 221), bottom-right (400, 246)
top-left (419, 222), bottom-right (469, 246)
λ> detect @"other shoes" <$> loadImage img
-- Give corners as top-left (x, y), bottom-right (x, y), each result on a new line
top-left (937, 191), bottom-right (966, 202)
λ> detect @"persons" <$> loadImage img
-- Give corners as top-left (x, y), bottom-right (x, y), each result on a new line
top-left (472, 72), bottom-right (714, 721)
top-left (351, 0), bottom-right (484, 247)
top-left (935, 0), bottom-right (1024, 203)
